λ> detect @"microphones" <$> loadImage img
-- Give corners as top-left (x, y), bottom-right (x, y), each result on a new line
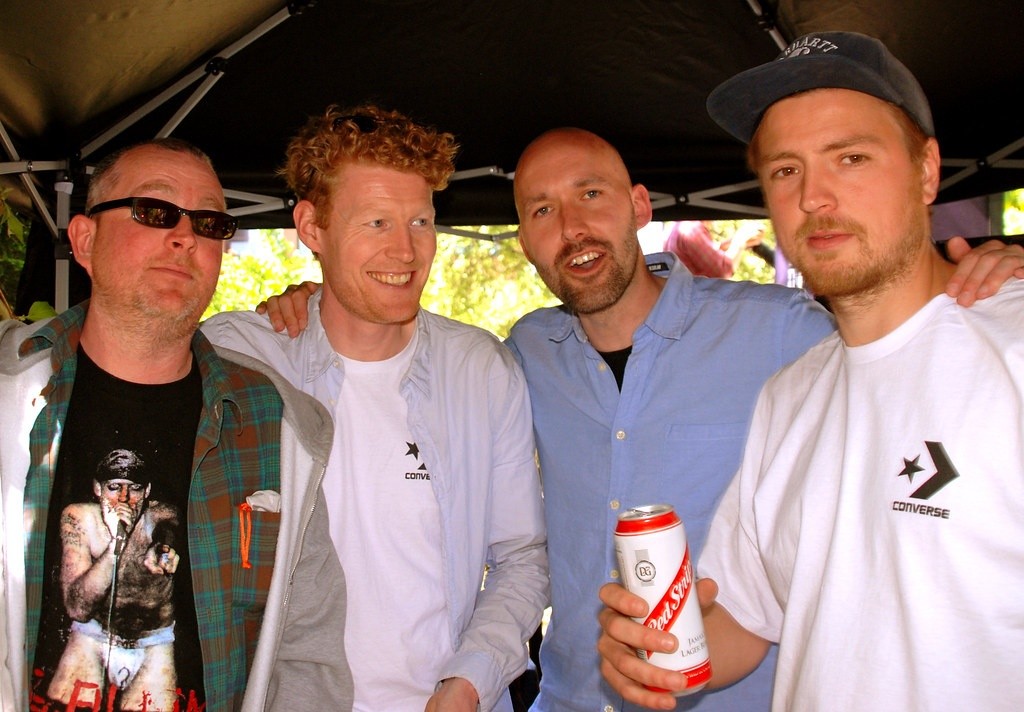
top-left (114, 520), bottom-right (128, 555)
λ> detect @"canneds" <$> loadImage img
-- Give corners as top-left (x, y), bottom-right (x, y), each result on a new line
top-left (615, 503), bottom-right (713, 696)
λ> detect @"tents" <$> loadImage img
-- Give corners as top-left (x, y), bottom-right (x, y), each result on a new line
top-left (0, 0), bottom-right (1024, 330)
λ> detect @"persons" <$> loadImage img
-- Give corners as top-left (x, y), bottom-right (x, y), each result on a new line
top-left (662, 219), bottom-right (766, 282)
top-left (0, 140), bottom-right (354, 712)
top-left (255, 127), bottom-right (1024, 712)
top-left (199, 104), bottom-right (551, 712)
top-left (597, 30), bottom-right (1024, 712)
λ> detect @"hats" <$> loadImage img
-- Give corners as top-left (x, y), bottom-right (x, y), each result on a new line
top-left (705, 31), bottom-right (935, 146)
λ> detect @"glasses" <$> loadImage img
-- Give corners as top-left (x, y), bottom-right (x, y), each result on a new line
top-left (87, 197), bottom-right (239, 240)
